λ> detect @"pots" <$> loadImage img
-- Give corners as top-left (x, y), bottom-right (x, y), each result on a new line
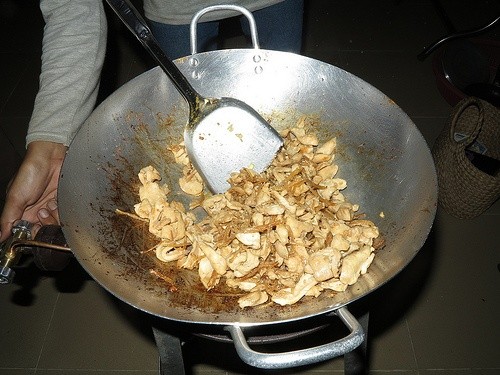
top-left (58, 6), bottom-right (439, 370)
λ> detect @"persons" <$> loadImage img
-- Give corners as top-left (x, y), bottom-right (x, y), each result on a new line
top-left (0, 0), bottom-right (304, 243)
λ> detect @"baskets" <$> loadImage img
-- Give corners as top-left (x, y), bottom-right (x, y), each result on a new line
top-left (434, 96), bottom-right (500, 223)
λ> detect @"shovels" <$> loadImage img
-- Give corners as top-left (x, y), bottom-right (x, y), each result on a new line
top-left (107, 0), bottom-right (284, 194)
